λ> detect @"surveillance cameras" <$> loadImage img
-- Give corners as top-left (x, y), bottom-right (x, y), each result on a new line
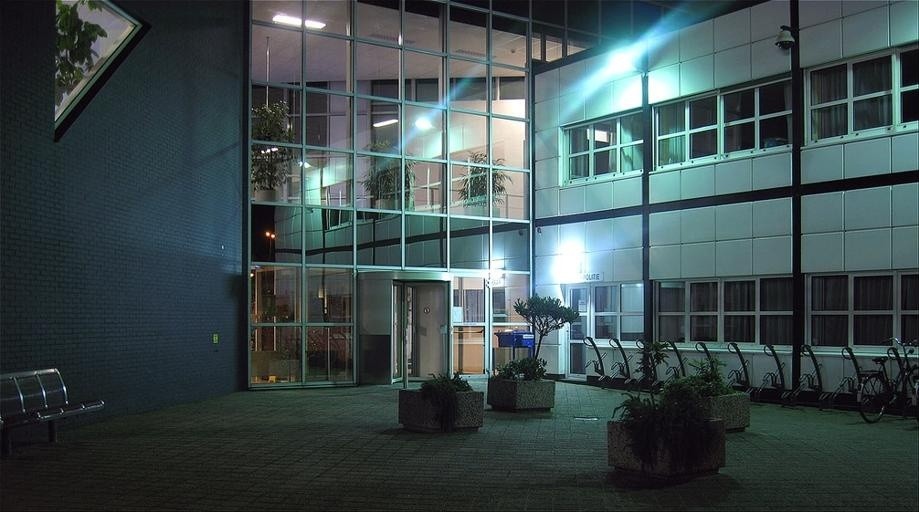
top-left (773, 30), bottom-right (796, 50)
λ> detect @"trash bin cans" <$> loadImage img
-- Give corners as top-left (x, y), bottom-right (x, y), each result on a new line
top-left (494, 329), bottom-right (534, 373)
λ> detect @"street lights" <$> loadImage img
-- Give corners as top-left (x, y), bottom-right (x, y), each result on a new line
top-left (265, 228), bottom-right (276, 264)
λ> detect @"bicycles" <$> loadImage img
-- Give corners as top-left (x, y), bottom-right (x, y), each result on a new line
top-left (856, 336), bottom-right (919, 424)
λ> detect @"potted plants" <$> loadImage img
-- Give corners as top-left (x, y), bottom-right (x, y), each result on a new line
top-left (399, 370), bottom-right (484, 433)
top-left (487, 293), bottom-right (579, 413)
top-left (250, 99), bottom-right (299, 202)
top-left (662, 355), bottom-right (751, 432)
top-left (608, 338), bottom-right (726, 480)
top-left (357, 137), bottom-right (415, 212)
top-left (461, 152), bottom-right (511, 219)
top-left (253, 308), bottom-right (300, 382)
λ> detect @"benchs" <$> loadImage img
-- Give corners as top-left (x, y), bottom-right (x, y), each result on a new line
top-left (1, 368), bottom-right (106, 451)
